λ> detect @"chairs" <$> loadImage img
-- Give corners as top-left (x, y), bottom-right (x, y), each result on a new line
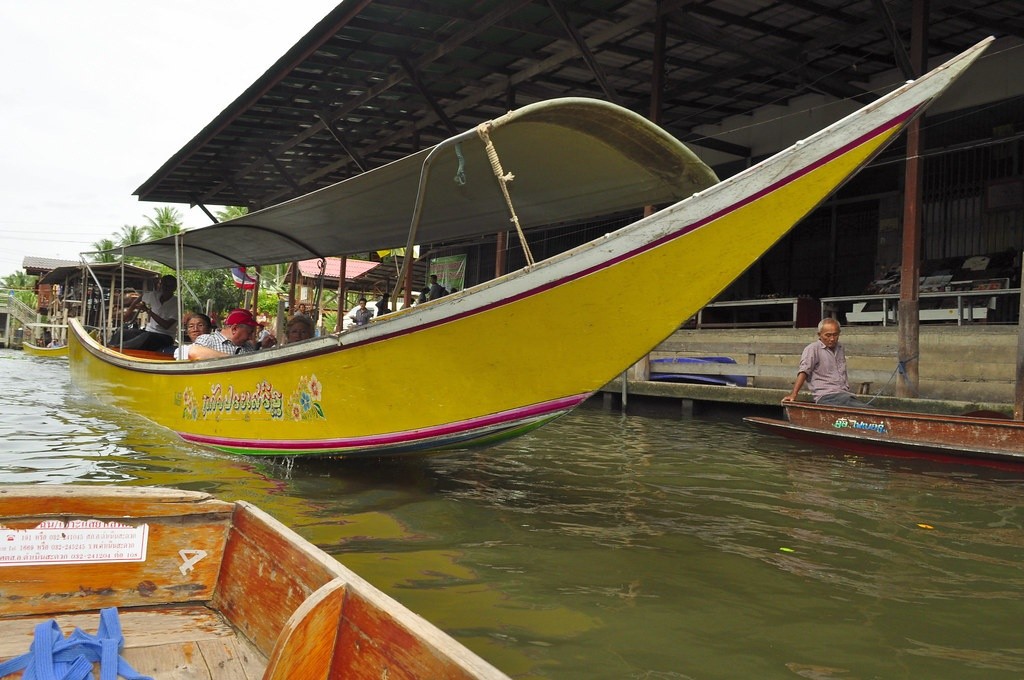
top-left (107, 327), bottom-right (180, 361)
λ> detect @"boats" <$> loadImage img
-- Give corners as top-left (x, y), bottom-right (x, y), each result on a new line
top-left (65, 36), bottom-right (999, 457)
top-left (742, 401), bottom-right (1024, 474)
top-left (20, 321), bottom-right (99, 357)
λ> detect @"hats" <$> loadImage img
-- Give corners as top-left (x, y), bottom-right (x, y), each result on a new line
top-left (222, 308), bottom-right (264, 328)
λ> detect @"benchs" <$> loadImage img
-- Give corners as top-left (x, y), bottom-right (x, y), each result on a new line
top-left (699, 287), bottom-right (1021, 325)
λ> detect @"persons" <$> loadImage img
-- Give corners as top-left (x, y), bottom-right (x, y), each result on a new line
top-left (429, 275), bottom-right (443, 300)
top-left (47, 338), bottom-right (61, 348)
top-left (442, 287), bottom-right (457, 296)
top-left (781, 318), bottom-right (874, 408)
top-left (419, 287), bottom-right (431, 305)
top-left (400, 298), bottom-right (414, 310)
top-left (353, 298), bottom-right (374, 326)
top-left (376, 293), bottom-right (391, 316)
top-left (294, 304), bottom-right (309, 318)
top-left (285, 315), bottom-right (315, 343)
top-left (110, 275), bottom-right (178, 351)
top-left (173, 309), bottom-right (270, 360)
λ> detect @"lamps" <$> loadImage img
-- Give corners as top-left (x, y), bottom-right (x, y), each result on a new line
top-left (664, 69), bottom-right (672, 89)
